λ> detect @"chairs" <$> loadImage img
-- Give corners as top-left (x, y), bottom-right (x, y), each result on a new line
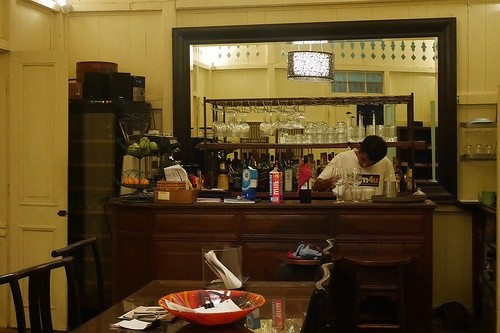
top-left (0, 256), bottom-right (74, 333)
top-left (51, 237), bottom-right (106, 331)
top-left (314, 238), bottom-right (337, 333)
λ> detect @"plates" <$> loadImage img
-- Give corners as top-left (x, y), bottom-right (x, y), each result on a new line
top-left (471, 117), bottom-right (495, 124)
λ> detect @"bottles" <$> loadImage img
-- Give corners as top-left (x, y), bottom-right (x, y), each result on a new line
top-left (217, 149), bottom-right (334, 204)
top-left (394, 156), bottom-right (415, 191)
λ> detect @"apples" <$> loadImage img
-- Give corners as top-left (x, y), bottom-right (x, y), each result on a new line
top-left (128, 137), bottom-right (158, 156)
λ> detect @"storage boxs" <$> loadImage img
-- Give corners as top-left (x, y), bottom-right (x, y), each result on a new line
top-left (242, 168), bottom-right (257, 200)
top-left (82, 72), bottom-right (130, 101)
top-left (155, 180), bottom-right (199, 203)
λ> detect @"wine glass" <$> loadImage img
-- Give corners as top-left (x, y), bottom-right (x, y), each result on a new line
top-left (331, 167), bottom-right (347, 204)
top-left (210, 107), bottom-right (305, 136)
top-left (347, 168), bottom-right (362, 202)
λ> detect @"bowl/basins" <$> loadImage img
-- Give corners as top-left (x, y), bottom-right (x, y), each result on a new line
top-left (159, 290), bottom-right (265, 327)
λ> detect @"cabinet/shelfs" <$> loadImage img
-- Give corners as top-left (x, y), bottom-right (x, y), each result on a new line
top-left (396, 126), bottom-right (438, 183)
top-left (66, 99), bottom-right (152, 310)
top-left (459, 121), bottom-right (496, 161)
top-left (197, 93), bottom-right (426, 189)
top-left (470, 202), bottom-right (497, 331)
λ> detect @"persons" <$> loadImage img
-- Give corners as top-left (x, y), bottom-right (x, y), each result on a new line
top-left (314, 134), bottom-right (397, 200)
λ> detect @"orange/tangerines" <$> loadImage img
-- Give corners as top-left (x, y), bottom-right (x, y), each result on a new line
top-left (124, 176), bottom-right (149, 184)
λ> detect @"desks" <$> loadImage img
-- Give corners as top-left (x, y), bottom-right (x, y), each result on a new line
top-left (66, 278), bottom-right (317, 333)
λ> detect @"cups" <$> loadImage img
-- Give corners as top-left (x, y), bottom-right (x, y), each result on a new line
top-left (201, 246), bottom-right (243, 289)
top-left (481, 191), bottom-right (496, 206)
top-left (464, 144), bottom-right (493, 158)
top-left (349, 187), bottom-right (376, 202)
top-left (383, 181), bottom-right (400, 198)
top-left (283, 121), bottom-right (397, 143)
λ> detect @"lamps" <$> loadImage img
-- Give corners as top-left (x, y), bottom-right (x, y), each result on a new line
top-left (286, 41), bottom-right (336, 84)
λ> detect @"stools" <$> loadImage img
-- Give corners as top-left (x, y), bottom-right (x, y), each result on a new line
top-left (283, 255), bottom-right (343, 266)
top-left (343, 255), bottom-right (412, 333)
